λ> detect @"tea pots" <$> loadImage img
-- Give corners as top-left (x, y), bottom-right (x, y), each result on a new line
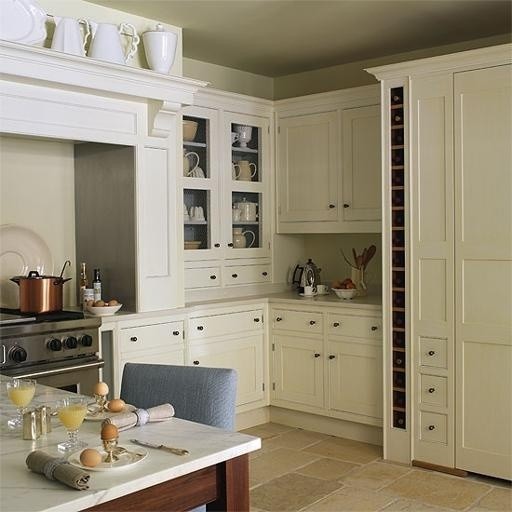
top-left (183, 148), bottom-right (200, 177)
top-left (50, 16), bottom-right (90, 56)
top-left (91, 21), bottom-right (139, 65)
top-left (234, 228), bottom-right (255, 248)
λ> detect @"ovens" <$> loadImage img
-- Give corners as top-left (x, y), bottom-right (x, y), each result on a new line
top-left (0, 329), bottom-right (104, 397)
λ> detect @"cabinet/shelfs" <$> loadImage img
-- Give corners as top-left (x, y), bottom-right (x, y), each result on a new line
top-left (186, 299), bottom-right (269, 431)
top-left (364, 42), bottom-right (512, 483)
top-left (269, 284), bottom-right (383, 449)
top-left (104, 312), bottom-right (187, 401)
top-left (181, 89), bottom-right (275, 300)
top-left (273, 83), bottom-right (382, 234)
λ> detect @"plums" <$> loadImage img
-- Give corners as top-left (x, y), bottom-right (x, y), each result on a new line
top-left (334, 278), bottom-right (356, 289)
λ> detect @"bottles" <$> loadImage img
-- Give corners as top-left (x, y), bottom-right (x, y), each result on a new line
top-left (232, 206), bottom-right (240, 222)
top-left (22, 405), bottom-right (52, 440)
top-left (233, 160), bottom-right (257, 182)
top-left (79, 262), bottom-right (102, 307)
top-left (189, 206), bottom-right (204, 218)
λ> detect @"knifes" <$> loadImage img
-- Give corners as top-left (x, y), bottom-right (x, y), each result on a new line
top-left (130, 438), bottom-right (189, 456)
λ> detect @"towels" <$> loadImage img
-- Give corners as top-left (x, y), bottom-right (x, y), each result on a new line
top-left (26, 450), bottom-right (90, 490)
top-left (101, 403), bottom-right (175, 430)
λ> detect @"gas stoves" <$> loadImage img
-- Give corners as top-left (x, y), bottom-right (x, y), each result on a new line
top-left (0, 305), bottom-right (102, 339)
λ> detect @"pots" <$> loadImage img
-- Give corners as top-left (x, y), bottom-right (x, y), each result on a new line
top-left (233, 197), bottom-right (259, 222)
top-left (9, 260), bottom-right (72, 314)
top-left (183, 120), bottom-right (199, 142)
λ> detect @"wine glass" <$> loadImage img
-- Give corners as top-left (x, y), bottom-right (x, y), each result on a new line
top-left (234, 125), bottom-right (252, 149)
top-left (6, 379), bottom-right (89, 450)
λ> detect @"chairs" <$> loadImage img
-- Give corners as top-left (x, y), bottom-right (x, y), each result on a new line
top-left (120, 363), bottom-right (236, 431)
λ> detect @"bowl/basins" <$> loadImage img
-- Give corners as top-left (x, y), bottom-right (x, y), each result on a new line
top-left (331, 288), bottom-right (357, 302)
top-left (184, 240), bottom-right (202, 249)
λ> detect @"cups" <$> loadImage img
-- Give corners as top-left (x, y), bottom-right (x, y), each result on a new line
top-left (304, 285), bottom-right (328, 294)
top-left (232, 132), bottom-right (239, 144)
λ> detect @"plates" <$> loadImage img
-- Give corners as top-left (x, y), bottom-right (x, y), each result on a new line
top-left (83, 401), bottom-right (136, 421)
top-left (68, 444), bottom-right (148, 471)
top-left (299, 292), bottom-right (330, 297)
top-left (86, 304), bottom-right (123, 315)
top-left (0, 223), bottom-right (55, 310)
top-left (0, 0), bottom-right (48, 44)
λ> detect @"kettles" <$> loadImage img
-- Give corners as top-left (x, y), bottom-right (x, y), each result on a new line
top-left (292, 259), bottom-right (321, 293)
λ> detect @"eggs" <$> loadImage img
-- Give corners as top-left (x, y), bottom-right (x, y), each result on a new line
top-left (109, 399), bottom-right (125, 411)
top-left (86, 299), bottom-right (118, 306)
top-left (101, 424), bottom-right (118, 440)
top-left (80, 449), bottom-right (101, 467)
top-left (95, 382), bottom-right (109, 395)
